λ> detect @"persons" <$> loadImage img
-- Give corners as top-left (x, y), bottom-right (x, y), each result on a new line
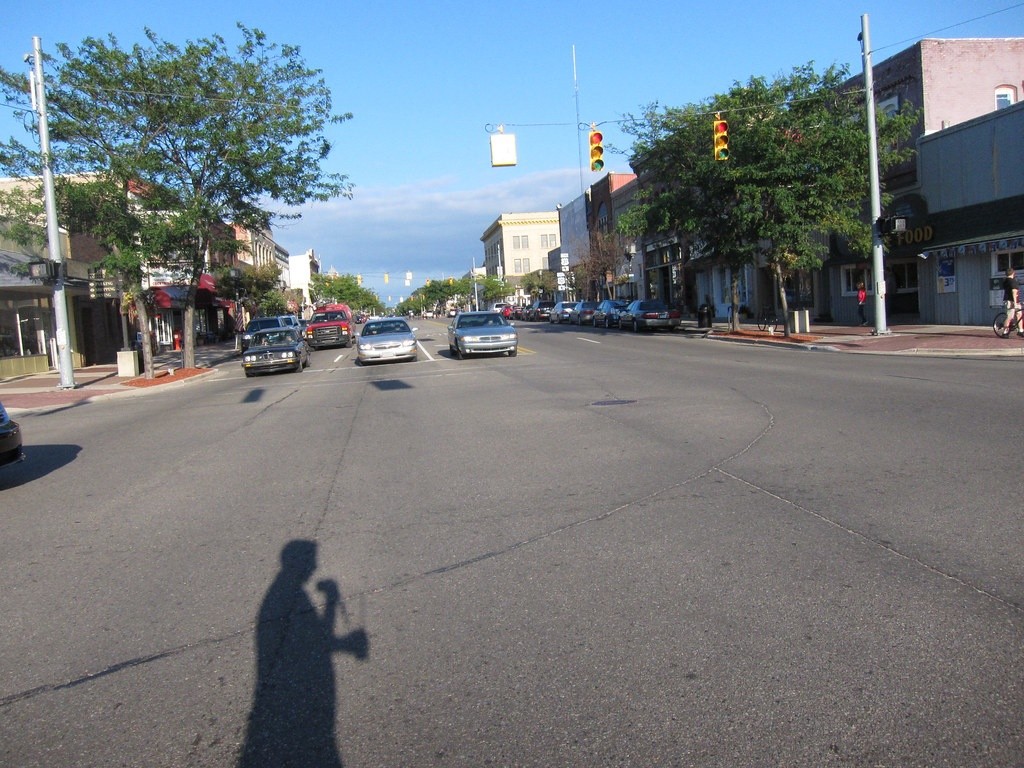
top-left (856, 281), bottom-right (869, 326)
top-left (483, 316), bottom-right (499, 326)
top-left (282, 333), bottom-right (295, 343)
top-left (885, 266), bottom-right (899, 316)
top-left (258, 337), bottom-right (272, 346)
top-left (367, 329), bottom-right (378, 335)
top-left (393, 324), bottom-right (402, 331)
top-left (1001, 268), bottom-right (1024, 338)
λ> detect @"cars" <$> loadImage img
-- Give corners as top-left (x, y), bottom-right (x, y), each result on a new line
top-left (355, 313), bottom-right (371, 324)
top-left (448, 309), bottom-right (457, 317)
top-left (354, 318), bottom-right (418, 363)
top-left (549, 301), bottom-right (580, 323)
top-left (592, 299), bottom-right (632, 329)
top-left (489, 302), bottom-right (533, 321)
top-left (447, 311), bottom-right (520, 359)
top-left (379, 312), bottom-right (403, 318)
top-left (425, 310), bottom-right (434, 319)
top-left (242, 327), bottom-right (313, 377)
top-left (568, 301), bottom-right (600, 325)
top-left (617, 299), bottom-right (681, 332)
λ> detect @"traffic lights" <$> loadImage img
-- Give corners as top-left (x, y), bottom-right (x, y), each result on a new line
top-left (878, 216), bottom-right (908, 236)
top-left (589, 127), bottom-right (606, 177)
top-left (29, 260), bottom-right (58, 281)
top-left (710, 115), bottom-right (735, 170)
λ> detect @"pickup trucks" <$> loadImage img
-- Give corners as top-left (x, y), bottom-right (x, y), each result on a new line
top-left (307, 302), bottom-right (355, 349)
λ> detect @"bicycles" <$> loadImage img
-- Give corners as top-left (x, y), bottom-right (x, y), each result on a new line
top-left (992, 302), bottom-right (1024, 337)
top-left (757, 306), bottom-right (778, 331)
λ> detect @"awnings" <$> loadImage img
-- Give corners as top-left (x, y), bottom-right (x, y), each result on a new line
top-left (197, 274), bottom-right (219, 294)
top-left (219, 301), bottom-right (231, 308)
top-left (149, 285), bottom-right (187, 309)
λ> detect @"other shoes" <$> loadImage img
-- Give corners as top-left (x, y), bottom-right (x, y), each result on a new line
top-left (1002, 334), bottom-right (1008, 337)
top-left (863, 321), bottom-right (869, 326)
top-left (859, 324), bottom-right (863, 327)
top-left (1017, 331), bottom-right (1024, 337)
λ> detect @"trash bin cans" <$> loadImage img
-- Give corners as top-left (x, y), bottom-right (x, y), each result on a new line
top-left (697, 306), bottom-right (712, 328)
top-left (789, 309), bottom-right (810, 333)
top-left (117, 351), bottom-right (140, 377)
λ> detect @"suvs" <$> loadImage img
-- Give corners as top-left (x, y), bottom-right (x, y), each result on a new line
top-left (241, 315), bottom-right (309, 352)
top-left (528, 301), bottom-right (556, 322)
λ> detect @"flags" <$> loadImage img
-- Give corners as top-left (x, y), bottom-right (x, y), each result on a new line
top-left (917, 218), bottom-right (1024, 259)
